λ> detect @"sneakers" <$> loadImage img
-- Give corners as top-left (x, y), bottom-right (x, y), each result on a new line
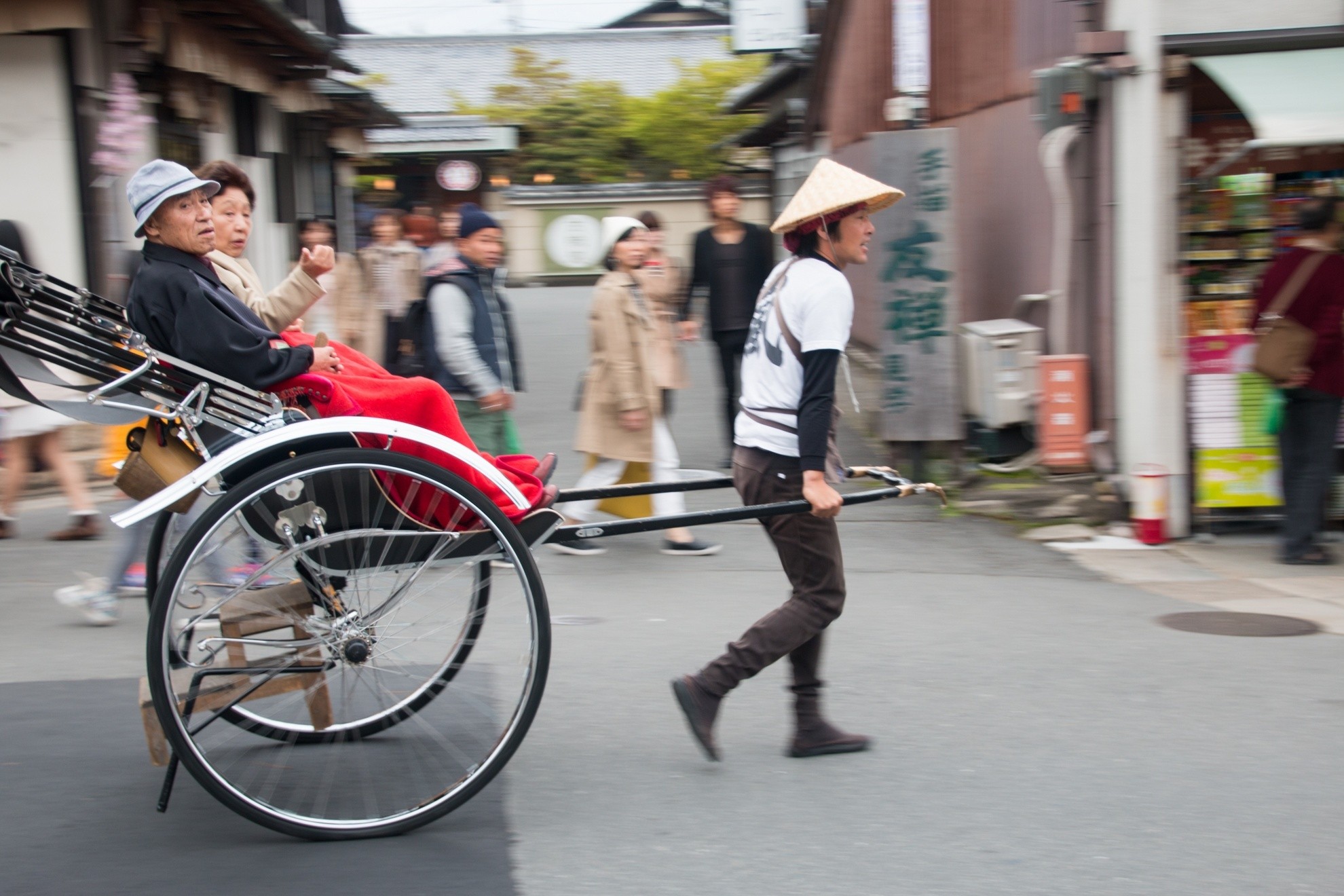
top-left (672, 675), bottom-right (720, 763)
top-left (549, 539), bottom-right (607, 556)
top-left (658, 537), bottom-right (723, 556)
top-left (792, 717), bottom-right (870, 758)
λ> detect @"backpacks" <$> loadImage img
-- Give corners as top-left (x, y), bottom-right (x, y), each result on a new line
top-left (391, 273), bottom-right (484, 378)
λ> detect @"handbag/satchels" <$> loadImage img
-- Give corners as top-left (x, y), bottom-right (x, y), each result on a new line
top-left (1250, 316), bottom-right (1318, 383)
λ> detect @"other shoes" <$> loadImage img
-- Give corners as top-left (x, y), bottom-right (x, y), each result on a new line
top-left (54, 584), bottom-right (119, 625)
top-left (1281, 549), bottom-right (1330, 565)
top-left (0, 512), bottom-right (19, 539)
top-left (46, 510), bottom-right (105, 541)
top-left (224, 563), bottom-right (276, 589)
top-left (116, 562), bottom-right (148, 590)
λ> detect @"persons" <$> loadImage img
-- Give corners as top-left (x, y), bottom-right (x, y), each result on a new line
top-left (189, 158), bottom-right (541, 521)
top-left (674, 176), bottom-right (778, 467)
top-left (1245, 193), bottom-right (1344, 565)
top-left (281, 188), bottom-right (476, 381)
top-left (426, 199), bottom-right (529, 569)
top-left (543, 214), bottom-right (726, 557)
top-left (123, 155), bottom-right (562, 533)
top-left (622, 209), bottom-right (700, 420)
top-left (0, 203), bottom-right (297, 628)
top-left (667, 157), bottom-right (872, 764)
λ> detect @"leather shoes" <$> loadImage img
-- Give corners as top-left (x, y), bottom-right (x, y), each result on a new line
top-left (538, 484), bottom-right (560, 509)
top-left (533, 452), bottom-right (558, 487)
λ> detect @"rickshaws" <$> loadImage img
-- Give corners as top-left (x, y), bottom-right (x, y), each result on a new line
top-left (0, 245), bottom-right (947, 845)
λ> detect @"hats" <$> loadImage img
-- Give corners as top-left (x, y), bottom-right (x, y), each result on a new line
top-left (595, 215), bottom-right (649, 265)
top-left (460, 202), bottom-right (501, 239)
top-left (769, 157), bottom-right (907, 236)
top-left (125, 158), bottom-right (222, 239)
